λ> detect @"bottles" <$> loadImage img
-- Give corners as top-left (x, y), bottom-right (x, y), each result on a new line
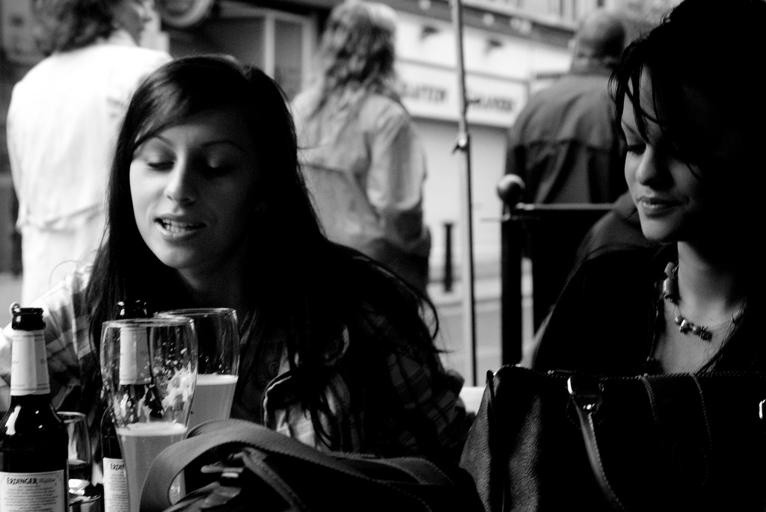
top-left (99, 299), bottom-right (170, 512)
top-left (0, 306), bottom-right (69, 512)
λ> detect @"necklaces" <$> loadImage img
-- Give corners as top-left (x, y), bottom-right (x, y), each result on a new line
top-left (660, 260), bottom-right (747, 341)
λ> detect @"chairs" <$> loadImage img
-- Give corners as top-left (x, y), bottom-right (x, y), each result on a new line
top-left (498, 173), bottom-right (612, 370)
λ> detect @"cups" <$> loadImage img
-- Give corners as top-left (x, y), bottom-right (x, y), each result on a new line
top-left (56, 411), bottom-right (92, 495)
top-left (99, 317), bottom-right (199, 512)
top-left (150, 307), bottom-right (240, 435)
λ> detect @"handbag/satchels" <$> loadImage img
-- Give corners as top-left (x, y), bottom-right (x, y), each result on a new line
top-left (139, 419), bottom-right (456, 511)
top-left (459, 363), bottom-right (720, 512)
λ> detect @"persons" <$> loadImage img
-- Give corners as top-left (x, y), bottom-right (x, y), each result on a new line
top-left (4, 0), bottom-right (172, 311)
top-left (0, 50), bottom-right (473, 512)
top-left (514, 2), bottom-right (765, 511)
top-left (503, 9), bottom-right (632, 327)
top-left (287, 0), bottom-right (434, 312)
